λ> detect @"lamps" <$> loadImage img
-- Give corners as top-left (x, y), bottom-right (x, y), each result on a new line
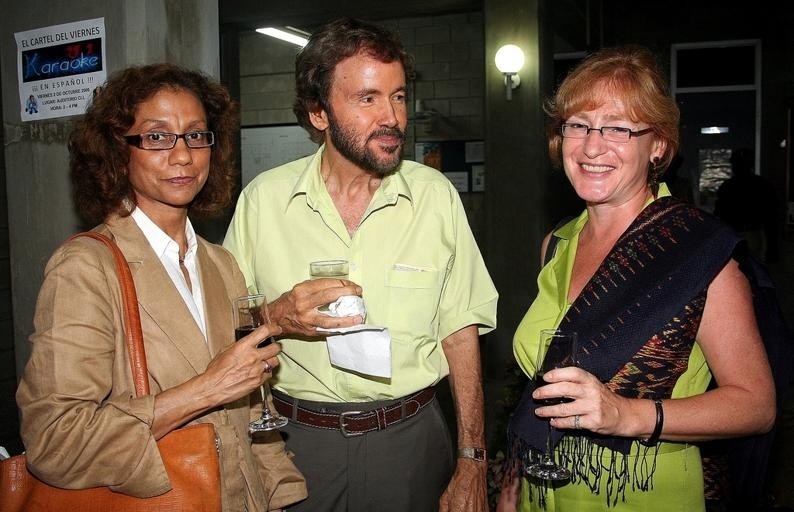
top-left (246, 19), bottom-right (314, 56)
top-left (495, 42), bottom-right (527, 101)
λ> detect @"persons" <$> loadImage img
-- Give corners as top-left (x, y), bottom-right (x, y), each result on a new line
top-left (491, 43), bottom-right (777, 512)
top-left (221, 14), bottom-right (501, 510)
top-left (15, 61), bottom-right (313, 511)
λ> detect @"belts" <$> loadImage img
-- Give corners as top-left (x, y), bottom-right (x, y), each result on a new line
top-left (273, 387), bottom-right (435, 438)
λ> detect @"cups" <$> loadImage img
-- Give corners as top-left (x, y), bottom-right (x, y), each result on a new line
top-left (310, 259), bottom-right (349, 313)
top-left (415, 99), bottom-right (424, 112)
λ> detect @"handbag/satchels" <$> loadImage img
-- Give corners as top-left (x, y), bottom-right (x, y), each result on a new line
top-left (0, 423), bottom-right (222, 511)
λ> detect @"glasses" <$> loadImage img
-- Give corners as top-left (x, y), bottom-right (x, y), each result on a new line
top-left (562, 123), bottom-right (652, 143)
top-left (123, 131), bottom-right (215, 150)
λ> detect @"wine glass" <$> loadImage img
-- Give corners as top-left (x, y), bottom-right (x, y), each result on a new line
top-left (524, 328), bottom-right (579, 480)
top-left (231, 294), bottom-right (289, 433)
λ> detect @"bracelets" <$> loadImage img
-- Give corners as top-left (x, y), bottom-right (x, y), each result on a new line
top-left (640, 397), bottom-right (665, 447)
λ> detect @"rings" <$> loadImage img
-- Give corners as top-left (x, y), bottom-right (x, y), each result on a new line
top-left (574, 413), bottom-right (583, 432)
top-left (263, 359), bottom-right (272, 371)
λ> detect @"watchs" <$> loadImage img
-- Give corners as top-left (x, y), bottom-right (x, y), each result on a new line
top-left (457, 448), bottom-right (488, 462)
